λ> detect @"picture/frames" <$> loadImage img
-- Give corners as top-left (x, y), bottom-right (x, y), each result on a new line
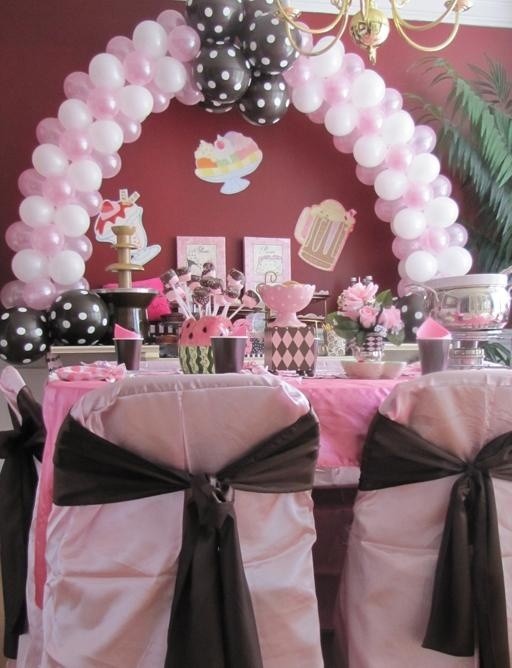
top-left (177, 236), bottom-right (226, 291)
top-left (243, 237), bottom-right (291, 305)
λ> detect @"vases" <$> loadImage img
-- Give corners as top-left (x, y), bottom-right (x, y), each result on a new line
top-left (350, 334), bottom-right (383, 363)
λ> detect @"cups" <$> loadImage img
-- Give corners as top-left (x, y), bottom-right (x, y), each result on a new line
top-left (211, 336), bottom-right (249, 373)
top-left (113, 338), bottom-right (144, 370)
top-left (416, 338), bottom-right (452, 373)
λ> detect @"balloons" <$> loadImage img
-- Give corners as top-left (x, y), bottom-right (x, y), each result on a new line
top-left (1, 53), bottom-right (155, 310)
top-left (106, 0), bottom-right (365, 128)
top-left (0, 307), bottom-right (52, 364)
top-left (396, 293), bottom-right (426, 344)
top-left (48, 289), bottom-right (109, 345)
top-left (326, 69), bottom-right (472, 296)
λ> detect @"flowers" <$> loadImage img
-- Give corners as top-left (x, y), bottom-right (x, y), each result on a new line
top-left (326, 281), bottom-right (405, 346)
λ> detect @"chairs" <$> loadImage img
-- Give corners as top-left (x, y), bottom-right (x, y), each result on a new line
top-left (0, 359), bottom-right (47, 657)
top-left (337, 369), bottom-right (512, 668)
top-left (17, 372), bottom-right (325, 668)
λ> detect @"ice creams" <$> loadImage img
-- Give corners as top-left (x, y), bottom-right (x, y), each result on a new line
top-left (194, 129), bottom-right (263, 195)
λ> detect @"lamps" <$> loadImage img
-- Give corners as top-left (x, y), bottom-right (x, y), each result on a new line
top-left (266, 0), bottom-right (473, 66)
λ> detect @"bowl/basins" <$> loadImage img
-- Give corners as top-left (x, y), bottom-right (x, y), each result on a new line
top-left (373, 348), bottom-right (421, 361)
top-left (384, 362), bottom-right (404, 379)
top-left (344, 358), bottom-right (380, 377)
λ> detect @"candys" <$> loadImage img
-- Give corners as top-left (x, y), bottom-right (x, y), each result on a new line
top-left (159, 259), bottom-right (260, 321)
